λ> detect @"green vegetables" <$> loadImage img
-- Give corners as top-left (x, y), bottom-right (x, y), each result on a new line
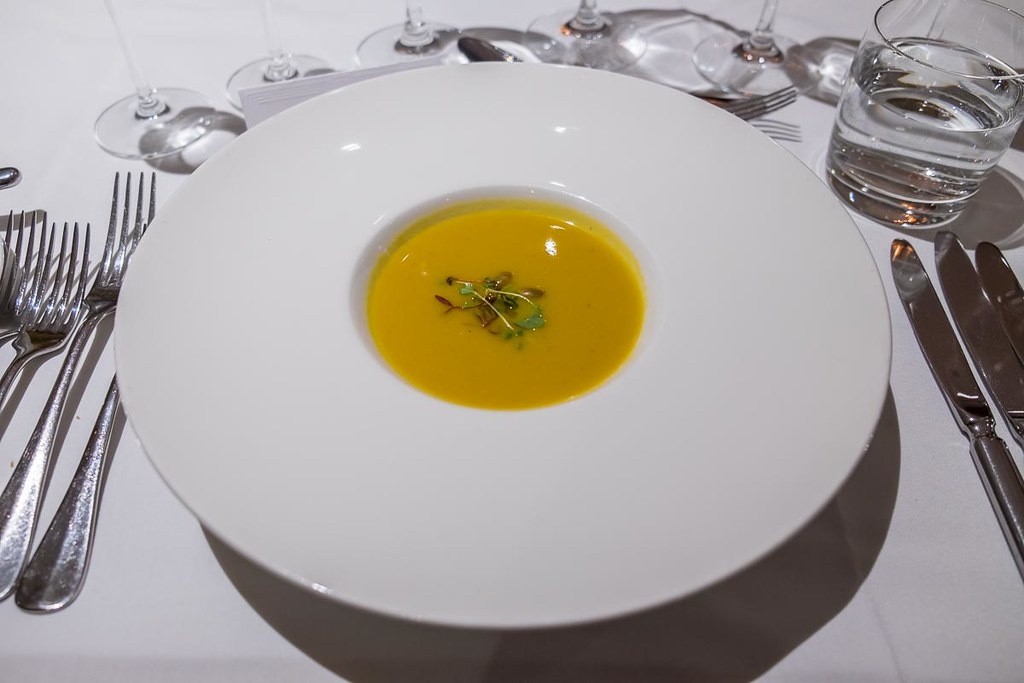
top-left (434, 276), bottom-right (545, 339)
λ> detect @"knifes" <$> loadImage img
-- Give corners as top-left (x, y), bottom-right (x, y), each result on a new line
top-left (890, 231), bottom-right (1023, 580)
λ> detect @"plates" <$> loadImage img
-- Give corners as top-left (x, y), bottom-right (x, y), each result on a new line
top-left (115, 62), bottom-right (892, 628)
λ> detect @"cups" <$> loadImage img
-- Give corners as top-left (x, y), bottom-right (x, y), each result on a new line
top-left (826, 1), bottom-right (1024, 230)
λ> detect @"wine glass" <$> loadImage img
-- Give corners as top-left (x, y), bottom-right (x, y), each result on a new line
top-left (226, 0), bottom-right (333, 111)
top-left (692, 0), bottom-right (814, 96)
top-left (525, 0), bottom-right (649, 70)
top-left (926, 0), bottom-right (960, 39)
top-left (356, 0), bottom-right (462, 66)
top-left (93, 0), bottom-right (216, 159)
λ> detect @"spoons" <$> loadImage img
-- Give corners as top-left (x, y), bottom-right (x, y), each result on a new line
top-left (458, 36), bottom-right (521, 63)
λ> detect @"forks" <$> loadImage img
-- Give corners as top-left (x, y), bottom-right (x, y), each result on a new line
top-left (1, 170), bottom-right (165, 615)
top-left (715, 86), bottom-right (799, 121)
